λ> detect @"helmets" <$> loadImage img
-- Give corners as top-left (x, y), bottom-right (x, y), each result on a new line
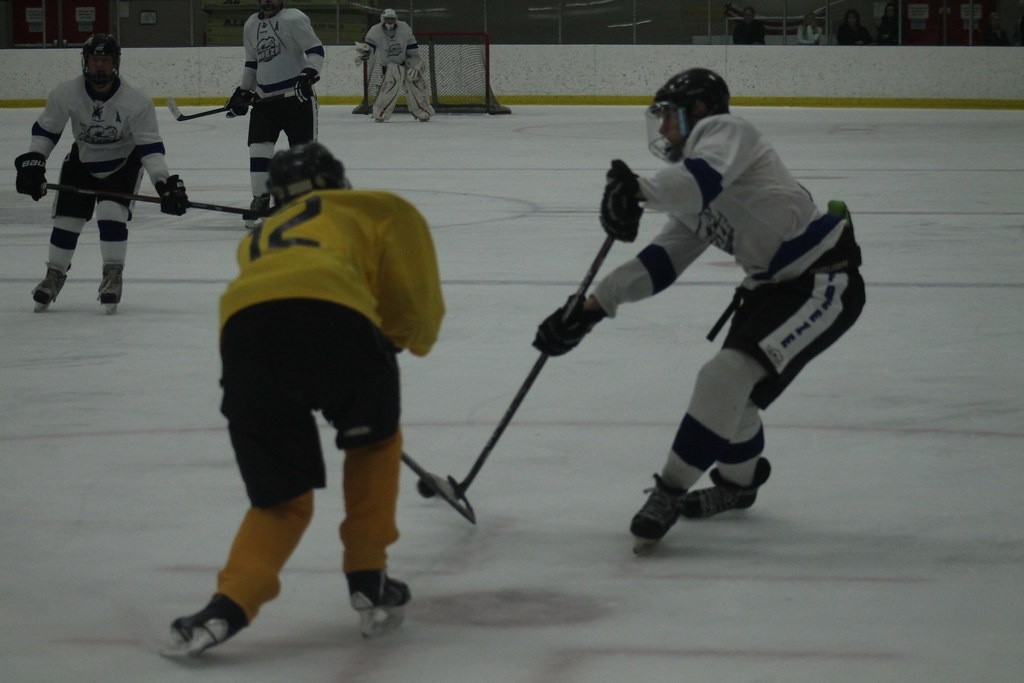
top-left (83, 33), bottom-right (121, 68)
top-left (380, 9), bottom-right (398, 25)
top-left (653, 68), bottom-right (729, 127)
top-left (267, 142), bottom-right (345, 206)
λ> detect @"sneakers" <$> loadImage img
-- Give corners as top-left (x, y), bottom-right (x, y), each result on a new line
top-left (150, 592), bottom-right (250, 658)
top-left (96, 263), bottom-right (124, 314)
top-left (684, 457), bottom-right (772, 519)
top-left (347, 569), bottom-right (412, 638)
top-left (31, 261), bottom-right (71, 313)
top-left (242, 193), bottom-right (270, 229)
top-left (629, 472), bottom-right (689, 554)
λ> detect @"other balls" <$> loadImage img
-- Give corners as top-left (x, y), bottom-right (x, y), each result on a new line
top-left (417, 478), bottom-right (436, 498)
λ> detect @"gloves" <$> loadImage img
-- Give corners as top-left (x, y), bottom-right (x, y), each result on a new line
top-left (294, 68), bottom-right (320, 104)
top-left (155, 175), bottom-right (191, 217)
top-left (531, 292), bottom-right (608, 357)
top-left (14, 151), bottom-right (48, 201)
top-left (599, 159), bottom-right (644, 243)
top-left (223, 86), bottom-right (255, 119)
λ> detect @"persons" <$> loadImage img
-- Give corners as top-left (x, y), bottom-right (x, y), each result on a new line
top-left (876, 3), bottom-right (910, 46)
top-left (222, 0), bottom-right (325, 222)
top-left (13, 32), bottom-right (190, 306)
top-left (797, 9), bottom-right (822, 45)
top-left (532, 67), bottom-right (867, 542)
top-left (732, 7), bottom-right (766, 45)
top-left (168, 143), bottom-right (445, 651)
top-left (836, 9), bottom-right (875, 46)
top-left (1014, 0), bottom-right (1024, 46)
top-left (356, 9), bottom-right (429, 123)
top-left (979, 11), bottom-right (1010, 46)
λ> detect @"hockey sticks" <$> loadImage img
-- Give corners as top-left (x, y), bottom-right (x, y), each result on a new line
top-left (46, 179), bottom-right (281, 219)
top-left (166, 90), bottom-right (314, 123)
top-left (400, 452), bottom-right (479, 527)
top-left (458, 235), bottom-right (617, 494)
top-left (362, 55), bottom-right (369, 115)
